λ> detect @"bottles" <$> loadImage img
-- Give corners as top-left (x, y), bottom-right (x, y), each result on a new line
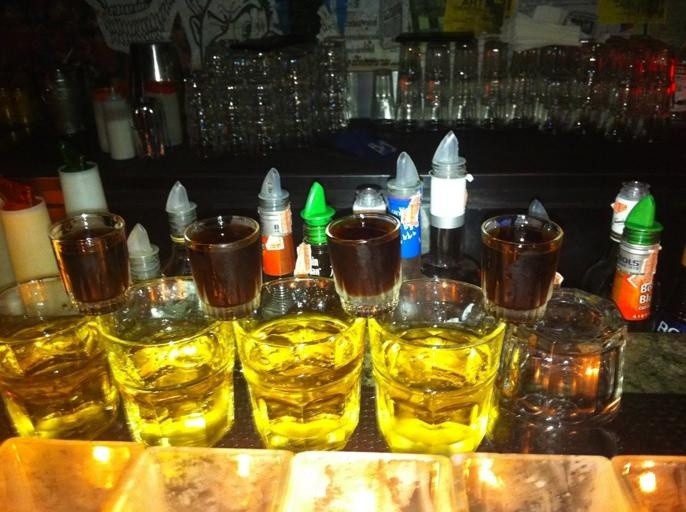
top-left (55, 126), bottom-right (109, 218)
top-left (3, 182), bottom-right (71, 317)
top-left (91, 80), bottom-right (184, 163)
top-left (610, 174), bottom-right (664, 330)
top-left (165, 182), bottom-right (206, 277)
top-left (300, 181), bottom-right (336, 277)
top-left (125, 224), bottom-right (164, 283)
top-left (424, 132), bottom-right (484, 285)
top-left (353, 185), bottom-right (388, 214)
top-left (256, 166), bottom-right (300, 318)
top-left (391, 152), bottom-right (428, 282)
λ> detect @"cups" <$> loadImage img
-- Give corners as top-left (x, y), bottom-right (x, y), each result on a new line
top-left (183, 37), bottom-right (356, 155)
top-left (369, 33), bottom-right (675, 130)
top-left (487, 288), bottom-right (629, 455)
top-left (49, 210), bottom-right (130, 316)
top-left (97, 274), bottom-right (237, 447)
top-left (181, 214), bottom-right (263, 320)
top-left (369, 276), bottom-right (509, 452)
top-left (0, 276), bottom-right (122, 442)
top-left (232, 273), bottom-right (368, 452)
top-left (325, 212), bottom-right (407, 316)
top-left (479, 211), bottom-right (567, 325)
